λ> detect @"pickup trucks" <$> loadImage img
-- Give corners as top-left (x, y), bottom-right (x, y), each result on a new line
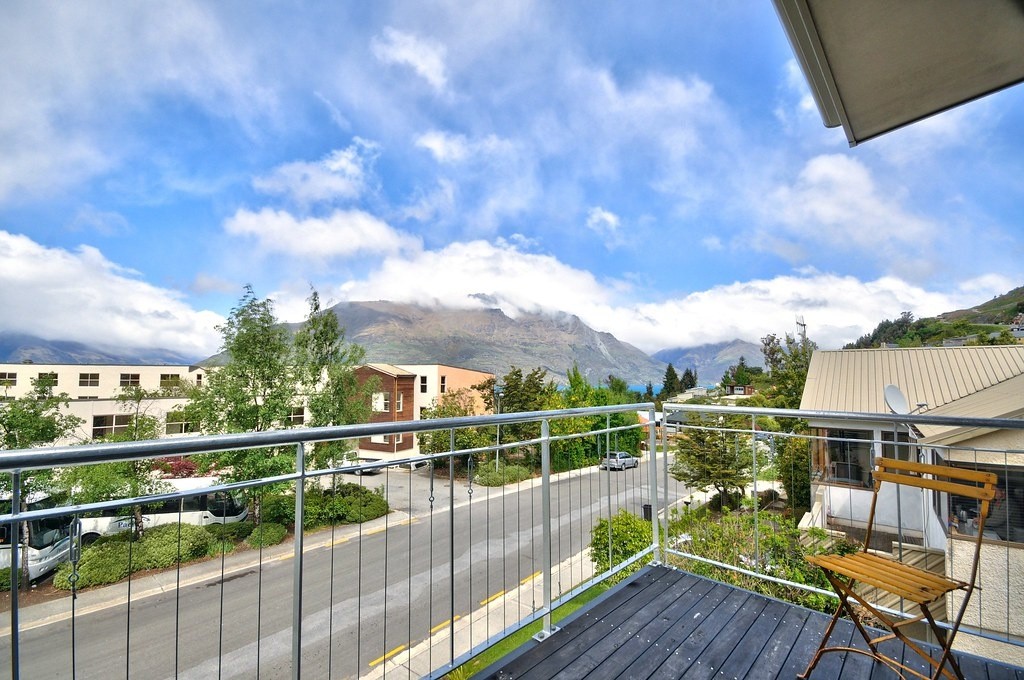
top-left (337, 449), bottom-right (384, 476)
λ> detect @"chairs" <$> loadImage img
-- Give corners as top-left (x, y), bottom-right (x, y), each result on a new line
top-left (798, 456), bottom-right (997, 680)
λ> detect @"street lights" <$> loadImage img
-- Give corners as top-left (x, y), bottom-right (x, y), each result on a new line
top-left (496, 392), bottom-right (505, 473)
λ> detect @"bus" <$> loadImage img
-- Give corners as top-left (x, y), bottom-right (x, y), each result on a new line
top-left (0, 486), bottom-right (79, 589)
top-left (69, 476), bottom-right (250, 547)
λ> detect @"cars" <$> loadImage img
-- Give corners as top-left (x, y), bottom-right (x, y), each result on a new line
top-left (602, 451), bottom-right (640, 471)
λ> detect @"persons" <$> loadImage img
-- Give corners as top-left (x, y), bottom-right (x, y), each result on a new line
top-left (973, 479), bottom-right (1021, 540)
